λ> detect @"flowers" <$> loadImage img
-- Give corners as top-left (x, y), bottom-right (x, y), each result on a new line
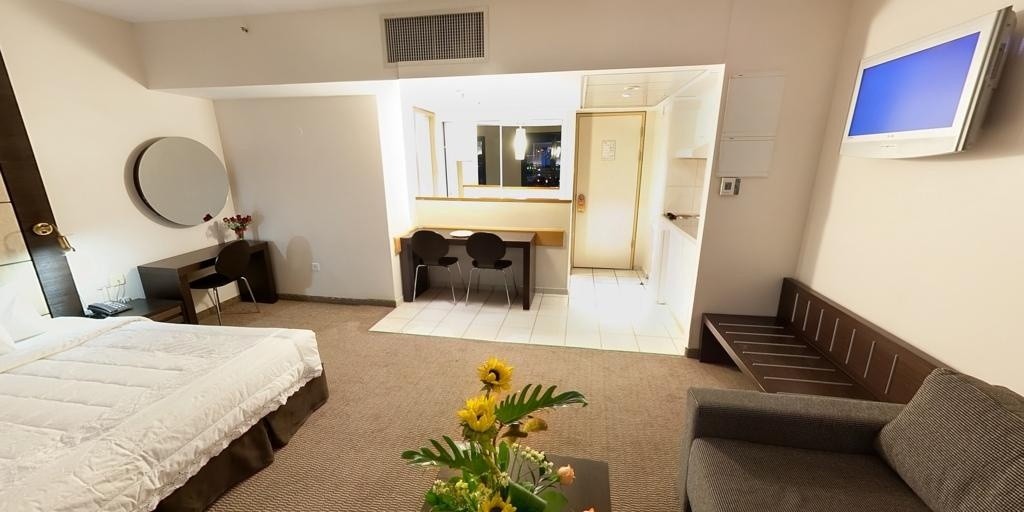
top-left (223, 214), bottom-right (254, 239)
top-left (401, 353), bottom-right (589, 512)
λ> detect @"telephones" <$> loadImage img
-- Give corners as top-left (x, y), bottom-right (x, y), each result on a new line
top-left (87, 300), bottom-right (132, 315)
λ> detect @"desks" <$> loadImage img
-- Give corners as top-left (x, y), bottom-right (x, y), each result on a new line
top-left (399, 227), bottom-right (537, 310)
top-left (137, 240), bottom-right (278, 325)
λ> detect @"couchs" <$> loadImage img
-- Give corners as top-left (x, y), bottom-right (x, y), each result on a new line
top-left (677, 367), bottom-right (1024, 512)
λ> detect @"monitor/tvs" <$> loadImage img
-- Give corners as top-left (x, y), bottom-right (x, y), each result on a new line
top-left (838, 4), bottom-right (1017, 159)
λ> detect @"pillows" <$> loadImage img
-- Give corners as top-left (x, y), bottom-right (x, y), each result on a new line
top-left (1, 280), bottom-right (50, 343)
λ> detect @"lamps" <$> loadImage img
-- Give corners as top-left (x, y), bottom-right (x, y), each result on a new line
top-left (510, 127), bottom-right (530, 161)
top-left (32, 221), bottom-right (77, 252)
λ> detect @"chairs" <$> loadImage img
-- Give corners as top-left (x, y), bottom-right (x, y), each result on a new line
top-left (189, 239), bottom-right (261, 326)
top-left (411, 230), bottom-right (465, 304)
top-left (464, 231), bottom-right (518, 309)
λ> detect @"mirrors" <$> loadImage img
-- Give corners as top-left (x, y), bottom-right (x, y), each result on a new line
top-left (138, 136), bottom-right (231, 225)
top-left (478, 125), bottom-right (561, 186)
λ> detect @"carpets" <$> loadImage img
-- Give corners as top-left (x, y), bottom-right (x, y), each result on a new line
top-left (197, 292), bottom-right (753, 512)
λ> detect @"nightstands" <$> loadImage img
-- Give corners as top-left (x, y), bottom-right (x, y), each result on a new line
top-left (84, 298), bottom-right (187, 326)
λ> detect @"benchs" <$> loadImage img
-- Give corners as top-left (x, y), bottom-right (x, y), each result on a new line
top-left (699, 275), bottom-right (951, 405)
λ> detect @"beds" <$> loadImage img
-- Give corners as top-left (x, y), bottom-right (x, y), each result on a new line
top-left (1, 316), bottom-right (328, 512)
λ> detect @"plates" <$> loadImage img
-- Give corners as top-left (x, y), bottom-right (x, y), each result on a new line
top-left (449, 230), bottom-right (474, 237)
